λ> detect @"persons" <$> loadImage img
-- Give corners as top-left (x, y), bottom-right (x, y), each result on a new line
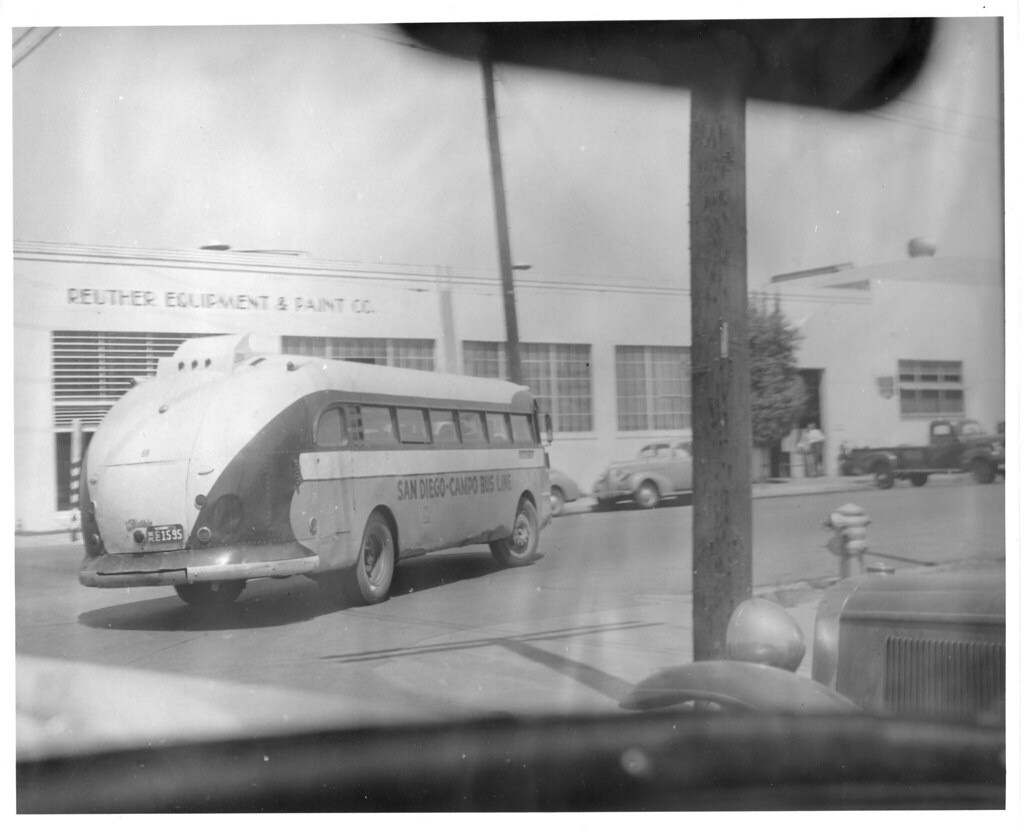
top-left (807, 422), bottom-right (825, 464)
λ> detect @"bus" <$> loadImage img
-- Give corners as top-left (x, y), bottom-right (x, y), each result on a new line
top-left (77, 331), bottom-right (553, 608)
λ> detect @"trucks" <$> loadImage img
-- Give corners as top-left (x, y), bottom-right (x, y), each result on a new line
top-left (837, 416), bottom-right (1005, 490)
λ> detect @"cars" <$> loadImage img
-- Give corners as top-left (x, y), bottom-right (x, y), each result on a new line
top-left (549, 468), bottom-right (579, 517)
top-left (593, 438), bottom-right (693, 508)
